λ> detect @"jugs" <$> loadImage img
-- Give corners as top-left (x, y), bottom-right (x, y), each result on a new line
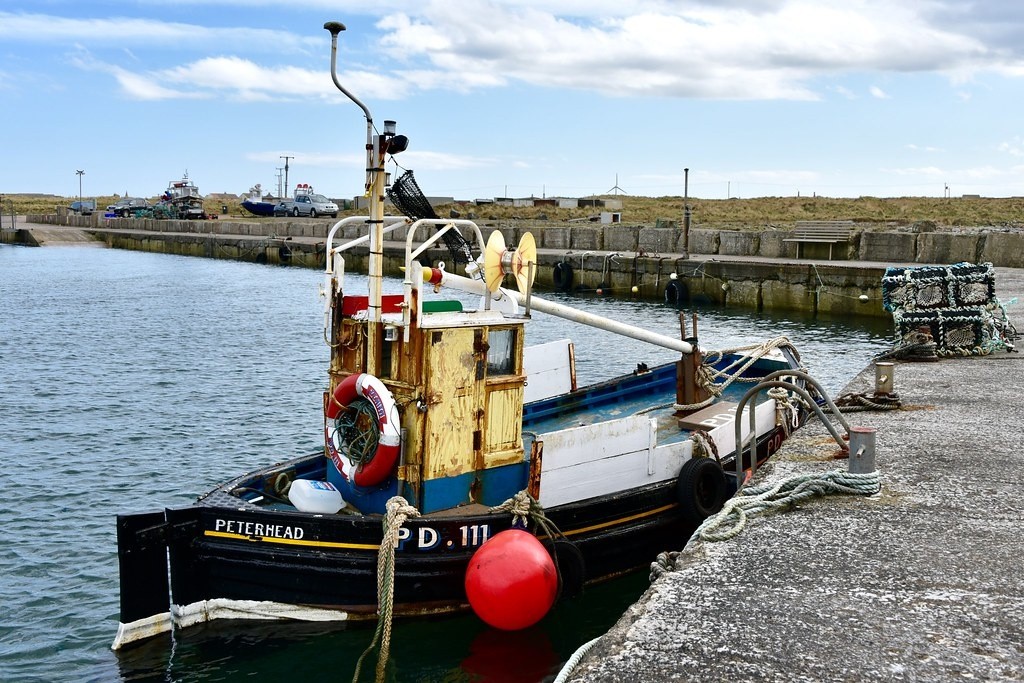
top-left (288, 479), bottom-right (347, 515)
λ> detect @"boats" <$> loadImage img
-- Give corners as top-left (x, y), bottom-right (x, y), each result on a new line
top-left (108, 20), bottom-right (810, 651)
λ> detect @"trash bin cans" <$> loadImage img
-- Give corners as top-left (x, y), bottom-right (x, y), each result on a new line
top-left (222, 206), bottom-right (227, 214)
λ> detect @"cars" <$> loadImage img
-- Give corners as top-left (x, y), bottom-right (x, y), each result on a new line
top-left (291, 193), bottom-right (339, 218)
top-left (273, 201), bottom-right (294, 217)
top-left (106, 198), bottom-right (153, 216)
